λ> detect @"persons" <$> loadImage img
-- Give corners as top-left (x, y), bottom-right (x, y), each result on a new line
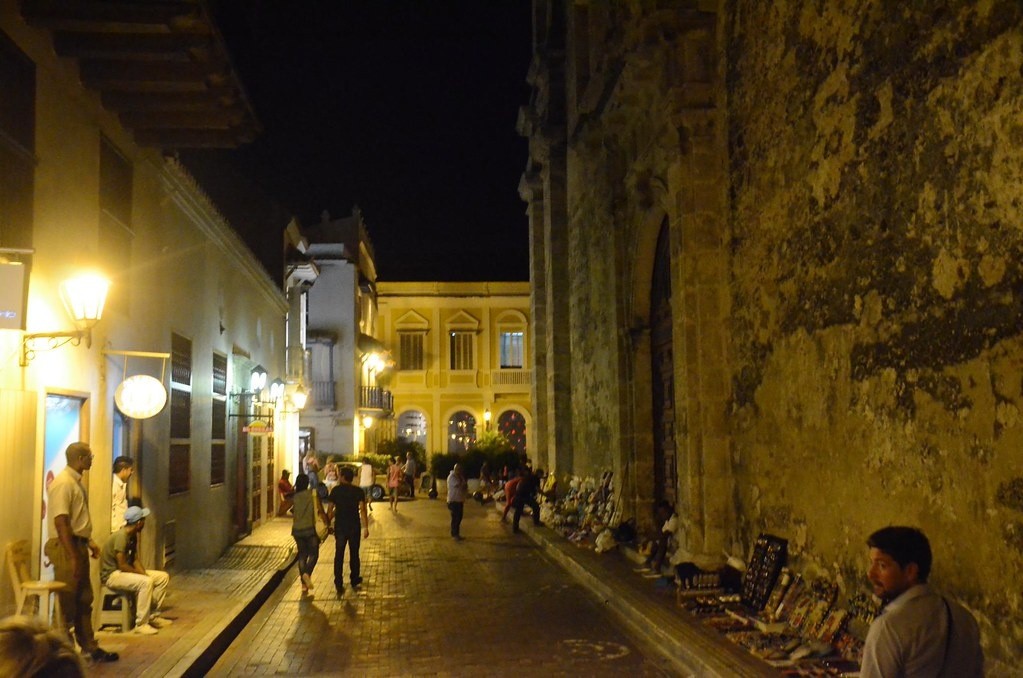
top-left (302, 449), bottom-right (319, 488)
top-left (99, 505), bottom-right (172, 635)
top-left (446, 464), bottom-right (468, 540)
top-left (111, 456), bottom-right (133, 534)
top-left (479, 459), bottom-right (544, 532)
top-left (291, 474), bottom-right (329, 593)
top-left (401, 451), bottom-right (417, 497)
top-left (357, 457), bottom-right (376, 511)
top-left (43, 441), bottom-right (118, 664)
top-left (385, 458), bottom-right (403, 512)
top-left (324, 456), bottom-right (339, 491)
top-left (278, 469), bottom-right (296, 514)
top-left (327, 467), bottom-right (369, 589)
top-left (0, 616), bottom-right (85, 678)
top-left (632, 500), bottom-right (680, 578)
top-left (394, 456), bottom-right (403, 468)
top-left (859, 526), bottom-right (984, 678)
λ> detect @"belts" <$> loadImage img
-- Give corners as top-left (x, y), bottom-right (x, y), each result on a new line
top-left (72, 534), bottom-right (88, 544)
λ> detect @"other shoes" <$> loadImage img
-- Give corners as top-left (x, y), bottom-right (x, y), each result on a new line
top-left (351, 577), bottom-right (363, 587)
top-left (92, 648), bottom-right (119, 662)
top-left (339, 585), bottom-right (346, 593)
top-left (302, 573), bottom-right (314, 590)
top-left (301, 584), bottom-right (308, 591)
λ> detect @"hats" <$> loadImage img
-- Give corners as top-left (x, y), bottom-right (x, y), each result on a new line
top-left (122, 506), bottom-right (149, 523)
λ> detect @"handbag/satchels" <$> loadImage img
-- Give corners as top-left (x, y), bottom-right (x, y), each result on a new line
top-left (316, 517), bottom-right (329, 543)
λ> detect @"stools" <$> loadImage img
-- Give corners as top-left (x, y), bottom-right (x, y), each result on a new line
top-left (17, 581), bottom-right (66, 628)
top-left (96, 586), bottom-right (130, 632)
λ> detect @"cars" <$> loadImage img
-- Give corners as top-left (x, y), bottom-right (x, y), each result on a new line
top-left (307, 461), bottom-right (390, 501)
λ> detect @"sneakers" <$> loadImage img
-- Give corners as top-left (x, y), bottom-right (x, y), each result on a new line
top-left (149, 617), bottom-right (173, 628)
top-left (134, 624), bottom-right (158, 635)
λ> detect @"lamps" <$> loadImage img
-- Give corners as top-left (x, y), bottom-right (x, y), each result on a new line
top-left (230, 364), bottom-right (267, 399)
top-left (247, 378), bottom-right (285, 408)
top-left (21, 271), bottom-right (113, 367)
top-left (279, 384), bottom-right (307, 413)
